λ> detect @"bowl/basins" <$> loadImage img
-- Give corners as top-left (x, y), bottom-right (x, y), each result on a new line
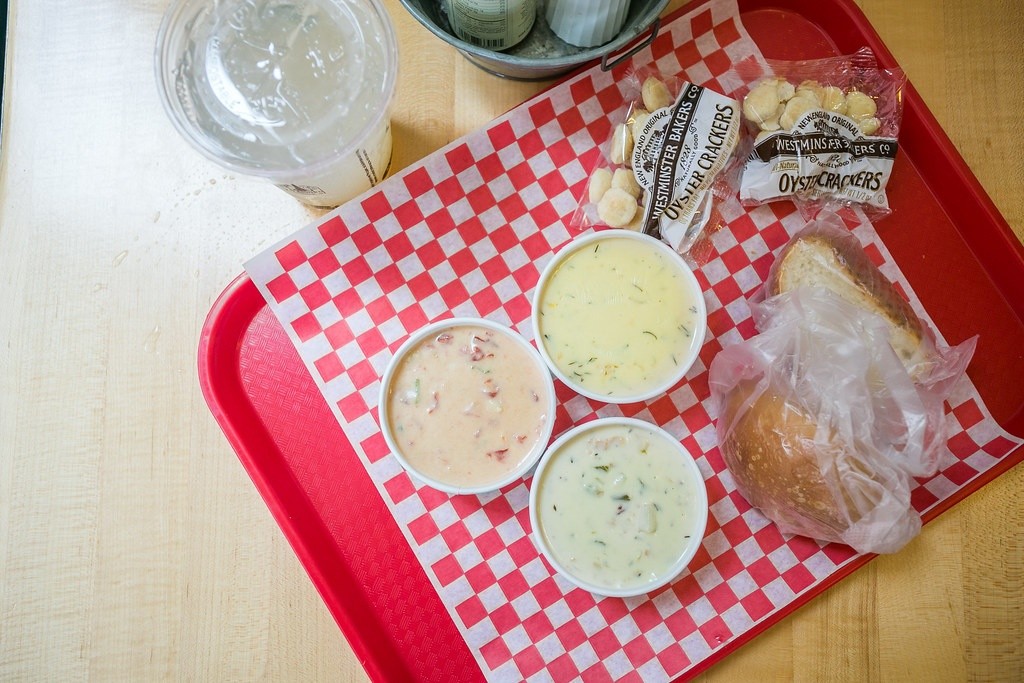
top-left (377, 318), bottom-right (556, 493)
top-left (398, 0), bottom-right (672, 82)
top-left (527, 416), bottom-right (708, 596)
top-left (532, 230), bottom-right (706, 403)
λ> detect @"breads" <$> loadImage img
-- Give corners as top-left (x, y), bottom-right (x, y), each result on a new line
top-left (776, 234), bottom-right (932, 390)
top-left (589, 75), bottom-right (680, 233)
top-left (743, 78), bottom-right (882, 143)
top-left (717, 380), bottom-right (888, 543)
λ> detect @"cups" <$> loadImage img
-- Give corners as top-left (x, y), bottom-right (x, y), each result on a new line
top-left (155, 0), bottom-right (400, 211)
